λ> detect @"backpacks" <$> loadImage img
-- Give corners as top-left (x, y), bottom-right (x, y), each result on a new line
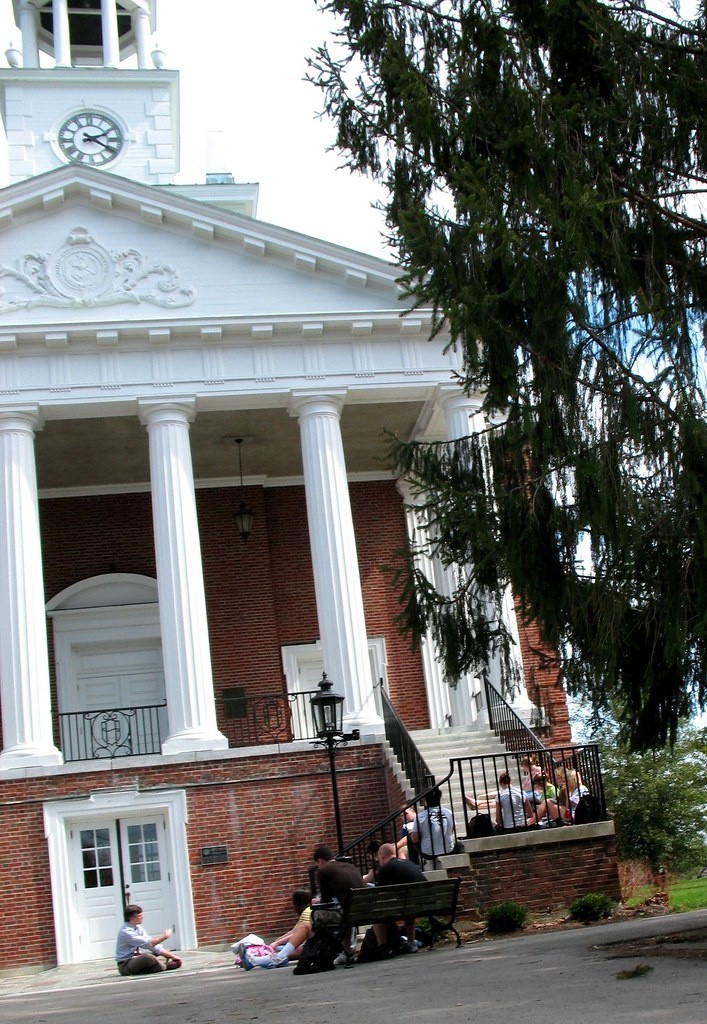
top-left (574, 794), bottom-right (600, 825)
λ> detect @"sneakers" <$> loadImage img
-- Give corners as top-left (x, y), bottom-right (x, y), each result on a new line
top-left (333, 954), bottom-right (351, 964)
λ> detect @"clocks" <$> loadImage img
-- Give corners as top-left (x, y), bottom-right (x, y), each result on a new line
top-left (43, 98), bottom-right (135, 170)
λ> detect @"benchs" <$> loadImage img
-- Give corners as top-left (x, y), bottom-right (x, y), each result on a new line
top-left (310, 876), bottom-right (463, 969)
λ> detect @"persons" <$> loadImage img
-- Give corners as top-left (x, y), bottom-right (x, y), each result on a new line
top-left (312, 846), bottom-right (372, 964)
top-left (374, 843), bottom-right (429, 960)
top-left (463, 754), bottom-right (590, 833)
top-left (115, 905), bottom-right (182, 976)
top-left (239, 786), bottom-right (454, 971)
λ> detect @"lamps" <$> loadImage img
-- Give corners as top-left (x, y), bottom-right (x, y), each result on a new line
top-left (233, 439), bottom-right (254, 541)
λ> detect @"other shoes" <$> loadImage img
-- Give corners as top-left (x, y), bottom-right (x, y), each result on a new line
top-left (406, 942), bottom-right (418, 952)
top-left (382, 945), bottom-right (393, 955)
top-left (242, 953), bottom-right (254, 969)
top-left (167, 960), bottom-right (183, 969)
top-left (261, 957), bottom-right (288, 967)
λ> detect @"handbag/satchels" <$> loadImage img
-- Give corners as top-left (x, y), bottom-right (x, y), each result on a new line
top-left (356, 923), bottom-right (401, 963)
top-left (456, 812), bottom-right (495, 840)
top-left (293, 934), bottom-right (337, 974)
top-left (230, 933), bottom-right (274, 965)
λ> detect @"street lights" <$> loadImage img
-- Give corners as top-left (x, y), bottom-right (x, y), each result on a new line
top-left (309, 670), bottom-right (347, 860)
